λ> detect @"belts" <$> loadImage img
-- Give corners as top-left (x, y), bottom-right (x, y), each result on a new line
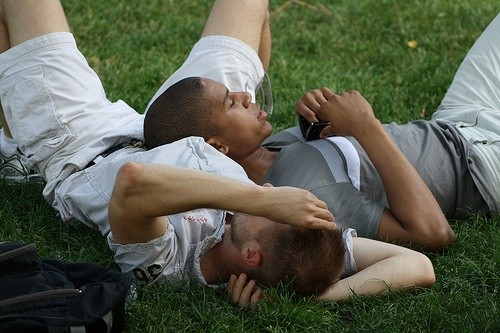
top-left (83, 138), bottom-right (136, 169)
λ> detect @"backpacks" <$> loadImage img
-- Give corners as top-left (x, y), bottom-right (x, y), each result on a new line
top-left (0, 241), bottom-right (130, 333)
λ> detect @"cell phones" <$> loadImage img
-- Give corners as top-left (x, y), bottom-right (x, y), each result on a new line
top-left (298, 112), bottom-right (334, 141)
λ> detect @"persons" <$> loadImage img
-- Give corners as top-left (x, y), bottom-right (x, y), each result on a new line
top-left (143, 12), bottom-right (500, 250)
top-left (0, 0), bottom-right (435, 311)
